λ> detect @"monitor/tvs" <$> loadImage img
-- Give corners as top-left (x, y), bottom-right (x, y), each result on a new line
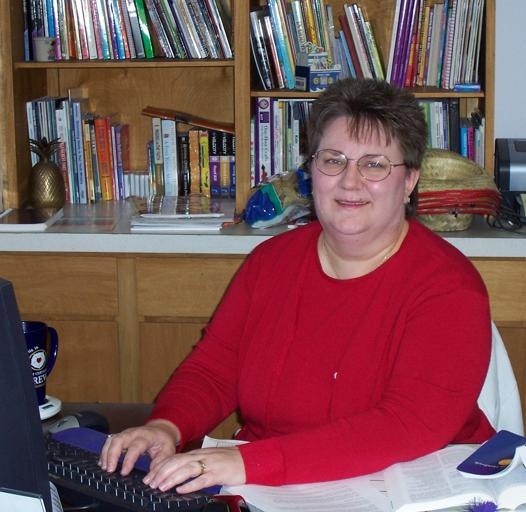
top-left (0, 277), bottom-right (51, 511)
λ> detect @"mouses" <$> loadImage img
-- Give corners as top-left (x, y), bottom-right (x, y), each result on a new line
top-left (48, 410), bottom-right (110, 433)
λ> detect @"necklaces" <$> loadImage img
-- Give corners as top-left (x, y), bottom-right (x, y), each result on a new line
top-left (322, 240), bottom-right (390, 279)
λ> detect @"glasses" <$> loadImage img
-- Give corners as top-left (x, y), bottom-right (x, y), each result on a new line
top-left (310, 147), bottom-right (406, 183)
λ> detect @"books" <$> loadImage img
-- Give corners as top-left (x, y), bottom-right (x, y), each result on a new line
top-left (384, 446), bottom-right (526, 511)
top-left (458, 430), bottom-right (526, 480)
top-left (25, 94), bottom-right (235, 231)
top-left (0, 208), bottom-right (63, 232)
top-left (418, 97), bottom-right (485, 171)
top-left (22, 1), bottom-right (234, 62)
top-left (251, 97), bottom-right (319, 188)
top-left (247, 1), bottom-right (484, 91)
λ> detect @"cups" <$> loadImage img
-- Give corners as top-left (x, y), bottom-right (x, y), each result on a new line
top-left (19, 319), bottom-right (61, 402)
top-left (33, 36), bottom-right (57, 62)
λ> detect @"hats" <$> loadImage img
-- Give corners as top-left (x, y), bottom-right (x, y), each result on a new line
top-left (414, 148), bottom-right (503, 231)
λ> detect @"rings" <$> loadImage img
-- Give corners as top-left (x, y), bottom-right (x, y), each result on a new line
top-left (196, 458), bottom-right (206, 474)
top-left (105, 433), bottom-right (117, 438)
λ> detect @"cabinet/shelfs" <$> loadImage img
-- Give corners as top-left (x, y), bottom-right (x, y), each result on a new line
top-left (0, 1), bottom-right (497, 220)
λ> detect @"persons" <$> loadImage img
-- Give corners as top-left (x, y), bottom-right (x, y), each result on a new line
top-left (96, 78), bottom-right (497, 496)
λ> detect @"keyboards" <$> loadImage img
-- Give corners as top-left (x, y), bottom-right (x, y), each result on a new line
top-left (44, 436), bottom-right (229, 512)
